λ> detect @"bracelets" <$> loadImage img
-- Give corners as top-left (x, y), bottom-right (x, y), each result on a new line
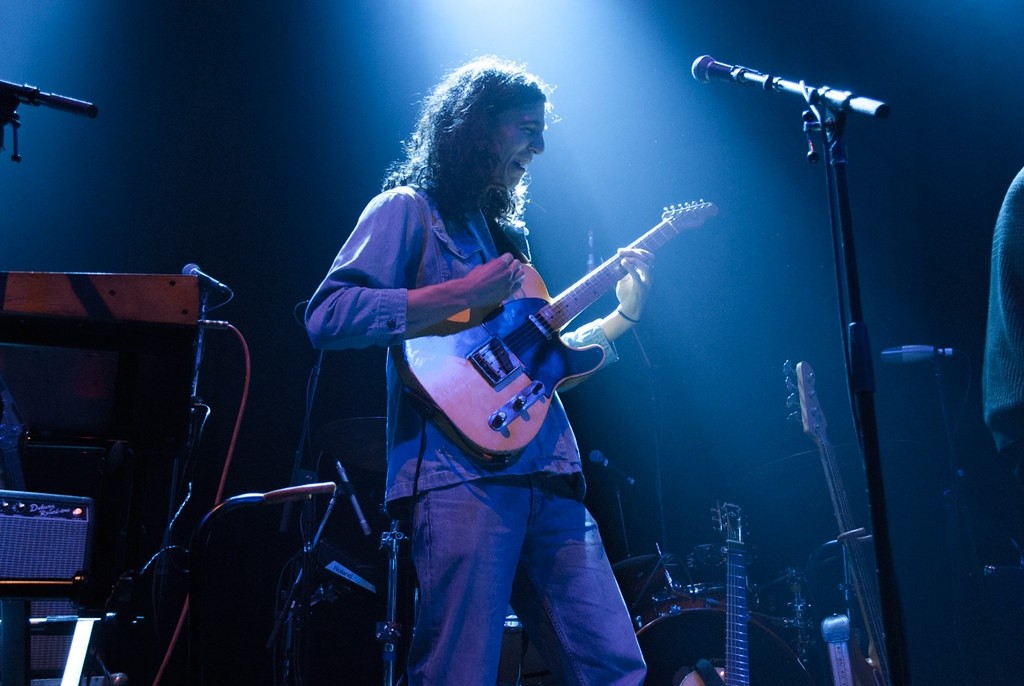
top-left (613, 302), bottom-right (642, 326)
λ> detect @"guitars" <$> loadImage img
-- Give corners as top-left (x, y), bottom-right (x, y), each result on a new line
top-left (395, 200), bottom-right (716, 466)
top-left (783, 359), bottom-right (892, 686)
top-left (671, 475), bottom-right (757, 686)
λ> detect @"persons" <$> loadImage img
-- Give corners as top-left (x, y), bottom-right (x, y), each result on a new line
top-left (975, 162), bottom-right (1023, 554)
top-left (672, 661), bottom-right (728, 686)
top-left (298, 53), bottom-right (661, 686)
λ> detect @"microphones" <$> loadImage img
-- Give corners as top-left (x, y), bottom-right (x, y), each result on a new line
top-left (589, 449), bottom-right (636, 486)
top-left (181, 264), bottom-right (232, 294)
top-left (334, 458), bottom-right (372, 536)
top-left (690, 55), bottom-right (758, 86)
top-left (880, 344), bottom-right (956, 361)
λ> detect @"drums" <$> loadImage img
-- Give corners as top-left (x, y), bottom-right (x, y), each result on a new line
top-left (636, 595), bottom-right (812, 685)
